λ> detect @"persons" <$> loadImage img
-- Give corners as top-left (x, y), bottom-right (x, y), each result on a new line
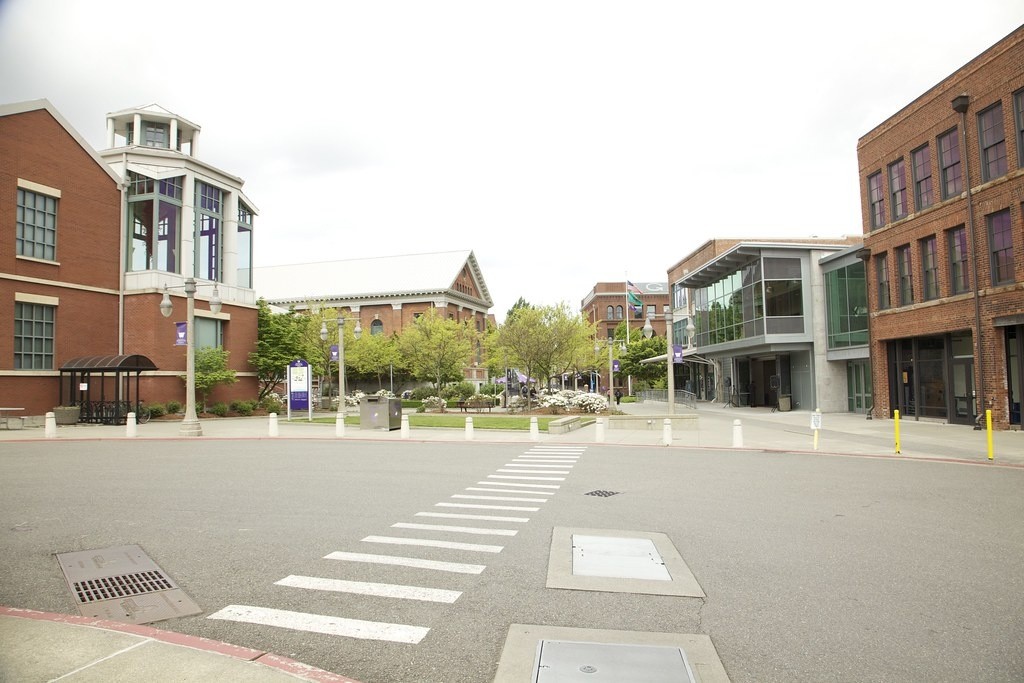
top-left (748, 380), bottom-right (757, 407)
top-left (607, 389), bottom-right (610, 399)
top-left (615, 388), bottom-right (623, 405)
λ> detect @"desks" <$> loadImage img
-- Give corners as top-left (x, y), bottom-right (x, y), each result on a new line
top-left (0, 407), bottom-right (25, 430)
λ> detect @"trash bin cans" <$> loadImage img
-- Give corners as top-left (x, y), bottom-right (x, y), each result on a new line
top-left (359, 395), bottom-right (402, 432)
top-left (778, 394), bottom-right (792, 412)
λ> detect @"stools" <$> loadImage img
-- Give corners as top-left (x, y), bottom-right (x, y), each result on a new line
top-left (16, 415), bottom-right (29, 431)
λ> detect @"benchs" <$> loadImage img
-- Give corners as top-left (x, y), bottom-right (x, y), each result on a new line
top-left (547, 416), bottom-right (582, 434)
top-left (456, 399), bottom-right (493, 412)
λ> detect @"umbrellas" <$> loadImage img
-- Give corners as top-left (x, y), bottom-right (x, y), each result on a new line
top-left (496, 368), bottom-right (537, 390)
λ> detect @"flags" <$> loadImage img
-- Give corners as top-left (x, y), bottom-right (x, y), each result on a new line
top-left (627, 292), bottom-right (644, 305)
top-left (628, 302), bottom-right (637, 312)
top-left (627, 280), bottom-right (644, 294)
